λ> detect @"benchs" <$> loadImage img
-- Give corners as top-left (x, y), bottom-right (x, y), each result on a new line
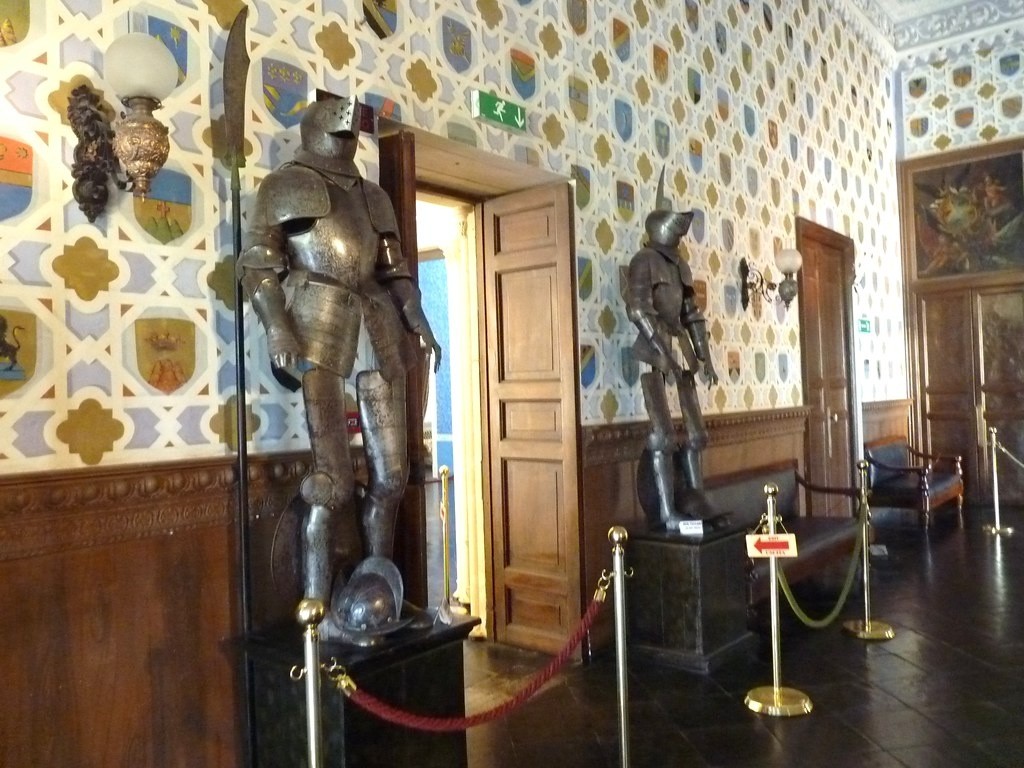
top-left (704, 459), bottom-right (872, 630)
top-left (865, 435), bottom-right (963, 532)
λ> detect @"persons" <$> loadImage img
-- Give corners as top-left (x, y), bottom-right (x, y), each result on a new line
top-left (235, 94), bottom-right (443, 649)
top-left (625, 210), bottom-right (738, 534)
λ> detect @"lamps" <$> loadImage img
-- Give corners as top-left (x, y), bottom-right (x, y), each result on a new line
top-left (741, 247), bottom-right (803, 311)
top-left (66, 32), bottom-right (179, 223)
